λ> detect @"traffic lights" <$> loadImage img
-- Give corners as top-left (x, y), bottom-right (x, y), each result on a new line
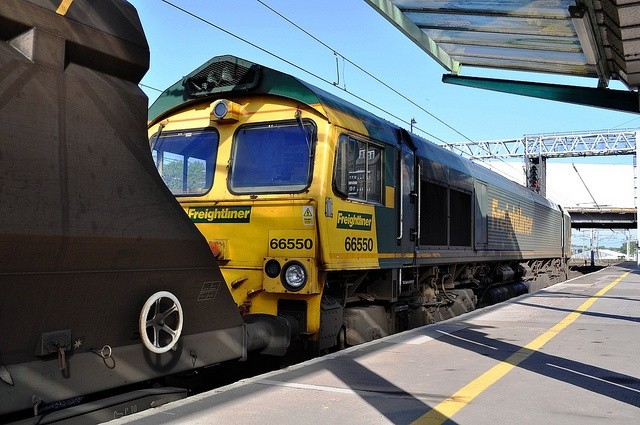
top-left (528, 165), bottom-right (540, 194)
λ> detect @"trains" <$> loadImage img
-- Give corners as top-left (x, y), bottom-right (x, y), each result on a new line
top-left (1, 1), bottom-right (575, 424)
